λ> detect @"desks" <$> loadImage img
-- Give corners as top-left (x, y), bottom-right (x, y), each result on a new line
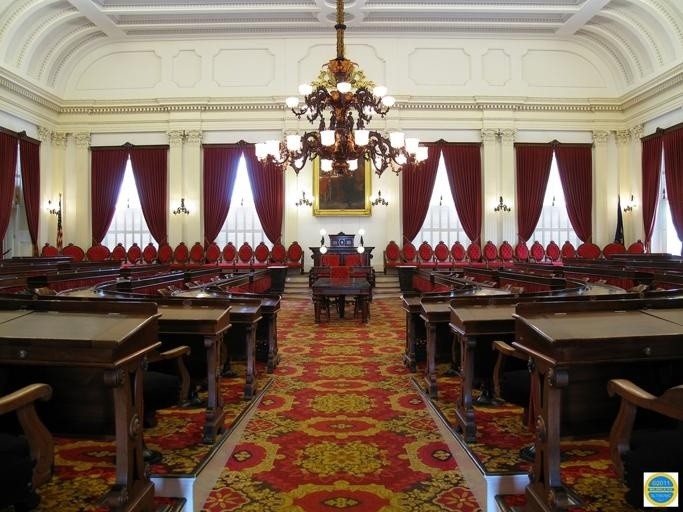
top-left (307, 245), bottom-right (377, 323)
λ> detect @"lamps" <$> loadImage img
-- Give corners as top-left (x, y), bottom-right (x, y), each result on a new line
top-left (310, 154), bottom-right (372, 220)
top-left (293, 190), bottom-right (313, 210)
top-left (47, 194), bottom-right (63, 214)
top-left (253, 1), bottom-right (434, 181)
top-left (369, 187), bottom-right (390, 208)
top-left (622, 194), bottom-right (636, 214)
top-left (492, 195), bottom-right (511, 215)
top-left (168, 196), bottom-right (195, 218)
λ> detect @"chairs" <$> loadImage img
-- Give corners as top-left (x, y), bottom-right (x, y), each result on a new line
top-left (379, 236), bottom-right (681, 512)
top-left (0, 238), bottom-right (308, 512)
